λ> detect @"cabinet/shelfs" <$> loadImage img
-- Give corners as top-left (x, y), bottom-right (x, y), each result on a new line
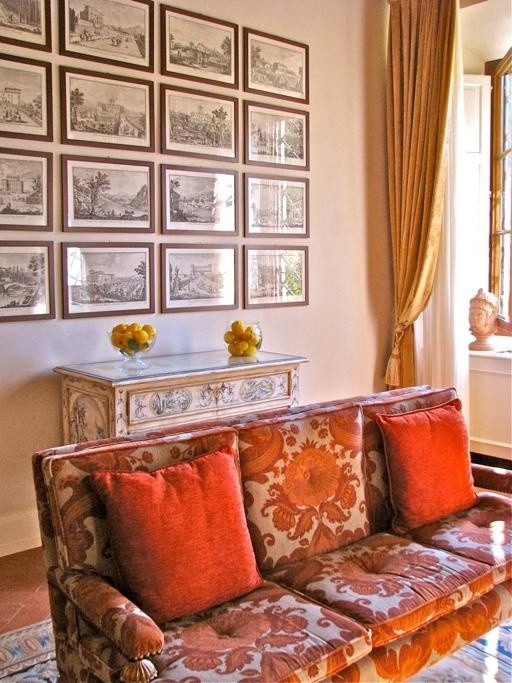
top-left (55, 348), bottom-right (311, 448)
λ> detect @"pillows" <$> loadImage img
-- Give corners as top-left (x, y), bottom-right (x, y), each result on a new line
top-left (85, 444), bottom-right (272, 625)
top-left (373, 395), bottom-right (483, 535)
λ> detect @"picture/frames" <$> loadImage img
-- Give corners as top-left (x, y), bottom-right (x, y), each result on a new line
top-left (1, 0), bottom-right (310, 321)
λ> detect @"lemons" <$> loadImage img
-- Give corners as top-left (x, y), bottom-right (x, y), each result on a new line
top-left (224, 321), bottom-right (260, 356)
top-left (111, 323), bottom-right (156, 354)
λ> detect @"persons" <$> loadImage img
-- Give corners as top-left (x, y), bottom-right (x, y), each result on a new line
top-left (468, 288), bottom-right (502, 326)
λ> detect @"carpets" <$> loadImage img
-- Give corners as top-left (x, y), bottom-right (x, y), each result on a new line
top-left (0, 600), bottom-right (511, 682)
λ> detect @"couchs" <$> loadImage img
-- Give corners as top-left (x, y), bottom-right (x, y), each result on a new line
top-left (29, 379), bottom-right (512, 682)
